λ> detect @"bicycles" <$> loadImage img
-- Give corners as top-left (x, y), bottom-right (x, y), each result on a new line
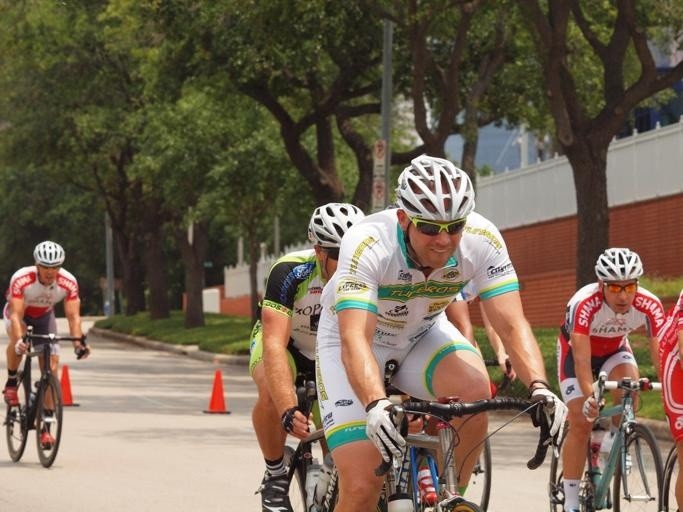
top-left (282, 372), bottom-right (387, 512)
top-left (456, 360), bottom-right (512, 511)
top-left (375, 359), bottom-right (554, 512)
top-left (4, 319), bottom-right (86, 470)
top-left (549, 373), bottom-right (683, 511)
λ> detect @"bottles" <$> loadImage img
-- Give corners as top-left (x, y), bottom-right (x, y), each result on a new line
top-left (27, 382), bottom-right (39, 408)
top-left (314, 453), bottom-right (334, 511)
top-left (596, 431), bottom-right (616, 470)
top-left (417, 464), bottom-right (437, 503)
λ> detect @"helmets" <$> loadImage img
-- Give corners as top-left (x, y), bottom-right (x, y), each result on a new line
top-left (308, 203), bottom-right (365, 248)
top-left (33, 241), bottom-right (65, 268)
top-left (594, 247), bottom-right (644, 282)
top-left (395, 153), bottom-right (475, 222)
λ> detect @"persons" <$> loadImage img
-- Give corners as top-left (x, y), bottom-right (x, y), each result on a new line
top-left (0, 238), bottom-right (92, 447)
top-left (312, 152), bottom-right (572, 511)
top-left (658, 287), bottom-right (682, 512)
top-left (556, 245), bottom-right (669, 512)
top-left (248, 202), bottom-right (369, 512)
top-left (400, 278), bottom-right (517, 507)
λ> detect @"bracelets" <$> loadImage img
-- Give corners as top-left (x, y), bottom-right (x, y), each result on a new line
top-left (527, 380), bottom-right (550, 397)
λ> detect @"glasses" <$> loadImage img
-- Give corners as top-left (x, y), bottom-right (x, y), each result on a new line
top-left (406, 214), bottom-right (467, 236)
top-left (319, 246), bottom-right (339, 262)
top-left (603, 280), bottom-right (639, 297)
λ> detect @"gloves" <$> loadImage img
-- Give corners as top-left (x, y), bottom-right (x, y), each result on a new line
top-left (365, 399), bottom-right (407, 463)
top-left (15, 339), bottom-right (23, 355)
top-left (282, 406), bottom-right (302, 433)
top-left (528, 388), bottom-right (568, 447)
top-left (582, 397), bottom-right (593, 416)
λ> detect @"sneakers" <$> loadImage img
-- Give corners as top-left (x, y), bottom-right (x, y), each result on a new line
top-left (41, 432), bottom-right (55, 446)
top-left (313, 484), bottom-right (327, 512)
top-left (255, 470), bottom-right (294, 512)
top-left (4, 386), bottom-right (18, 406)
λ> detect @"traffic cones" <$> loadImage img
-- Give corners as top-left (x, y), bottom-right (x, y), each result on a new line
top-left (52, 364), bottom-right (81, 407)
top-left (199, 368), bottom-right (234, 417)
